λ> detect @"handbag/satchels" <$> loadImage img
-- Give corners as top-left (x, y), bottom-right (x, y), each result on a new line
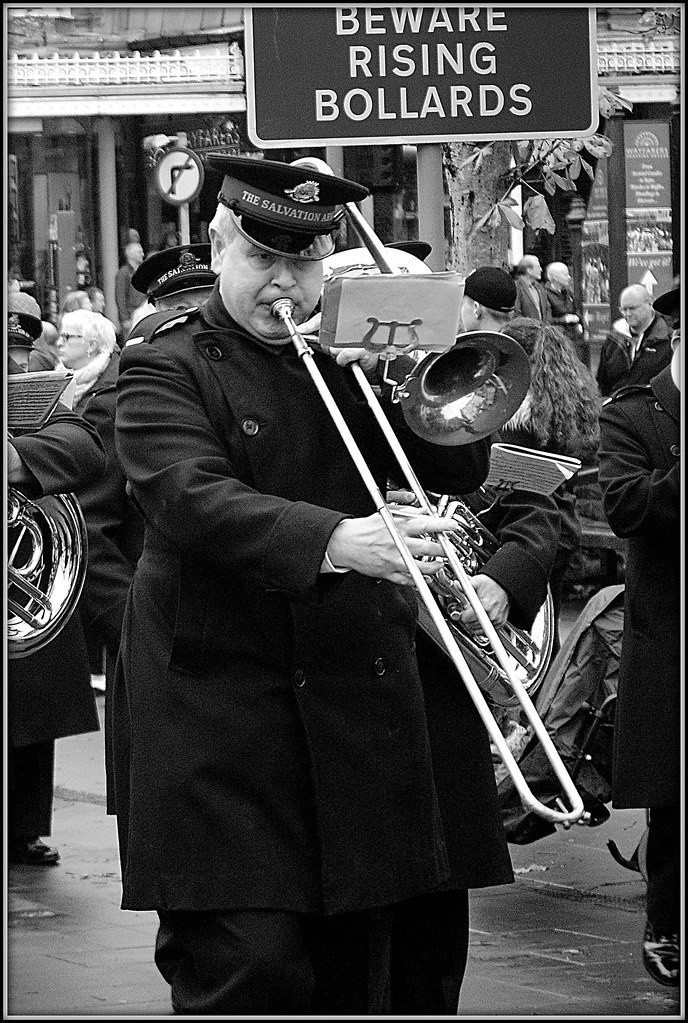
top-left (493, 583), bottom-right (628, 846)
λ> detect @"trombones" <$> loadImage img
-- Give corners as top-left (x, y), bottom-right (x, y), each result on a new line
top-left (269, 157), bottom-right (591, 830)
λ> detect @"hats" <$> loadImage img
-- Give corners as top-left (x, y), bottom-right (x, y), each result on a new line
top-left (386, 240), bottom-right (432, 265)
top-left (8, 291), bottom-right (42, 339)
top-left (652, 287), bottom-right (680, 319)
top-left (464, 265), bottom-right (519, 313)
top-left (8, 312), bottom-right (42, 349)
top-left (130, 242), bottom-right (219, 304)
top-left (205, 152), bottom-right (369, 260)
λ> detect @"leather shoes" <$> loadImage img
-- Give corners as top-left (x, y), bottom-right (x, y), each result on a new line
top-left (7, 836), bottom-right (60, 864)
top-left (642, 921), bottom-right (680, 986)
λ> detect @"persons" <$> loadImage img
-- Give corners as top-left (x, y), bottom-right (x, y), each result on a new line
top-left (5, 311), bottom-right (109, 863)
top-left (6, 228), bottom-right (598, 862)
top-left (105, 153), bottom-right (561, 1015)
top-left (595, 291), bottom-right (681, 984)
top-left (597, 284), bottom-right (672, 398)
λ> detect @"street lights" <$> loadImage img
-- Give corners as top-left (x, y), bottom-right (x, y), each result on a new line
top-left (144, 131), bottom-right (191, 247)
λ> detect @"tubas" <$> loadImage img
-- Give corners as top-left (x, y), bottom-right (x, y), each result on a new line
top-left (7, 430), bottom-right (89, 660)
top-left (386, 482), bottom-right (555, 708)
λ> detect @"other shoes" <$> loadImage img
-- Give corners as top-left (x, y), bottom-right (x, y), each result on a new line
top-left (91, 673), bottom-right (106, 692)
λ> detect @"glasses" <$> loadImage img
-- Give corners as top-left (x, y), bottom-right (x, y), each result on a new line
top-left (61, 333), bottom-right (83, 342)
top-left (617, 299), bottom-right (649, 314)
